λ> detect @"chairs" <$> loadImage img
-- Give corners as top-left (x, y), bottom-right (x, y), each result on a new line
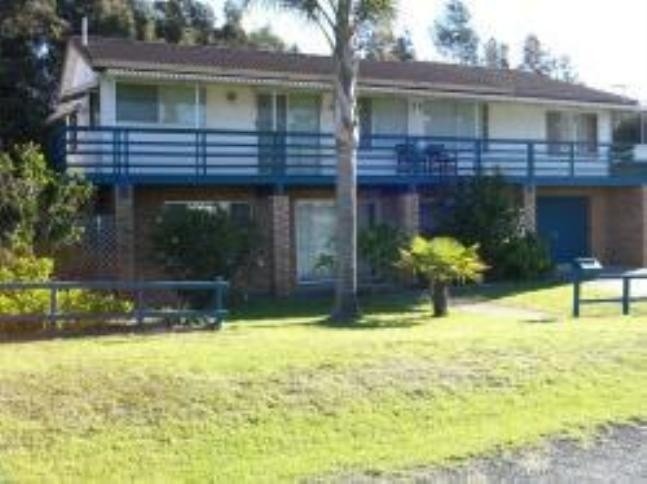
top-left (396, 143), bottom-right (455, 176)
